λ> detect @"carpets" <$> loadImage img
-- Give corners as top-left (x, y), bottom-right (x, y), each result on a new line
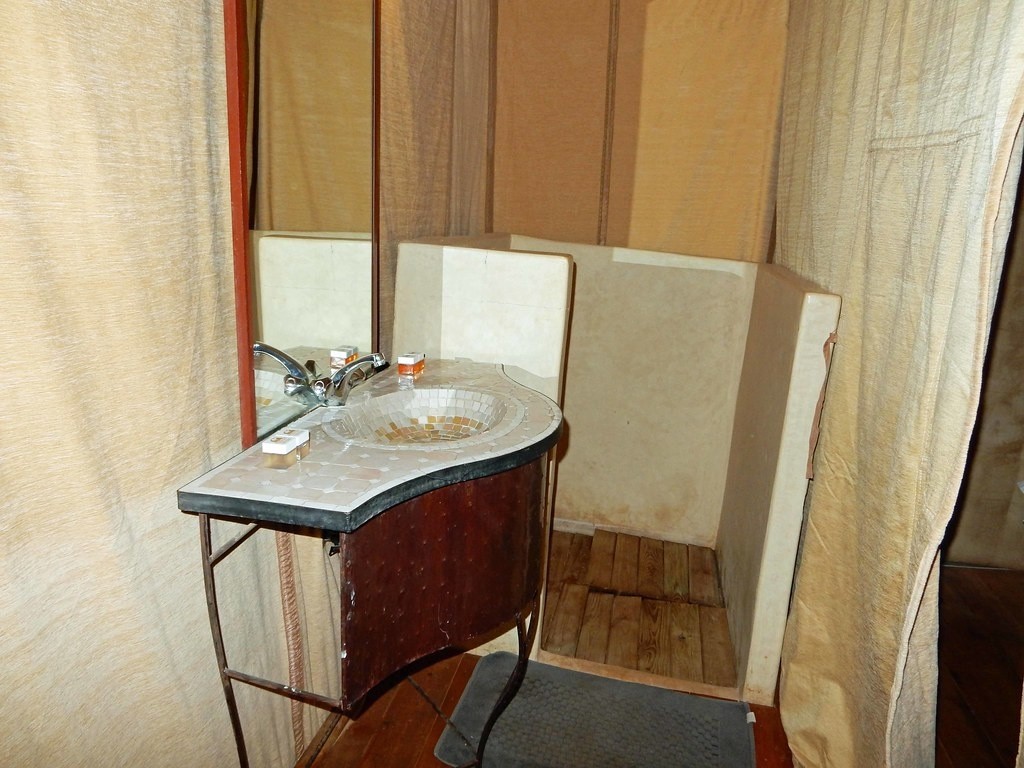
top-left (434, 650), bottom-right (756, 768)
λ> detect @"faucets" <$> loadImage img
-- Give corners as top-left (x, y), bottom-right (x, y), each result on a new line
top-left (253, 341), bottom-right (318, 406)
top-left (325, 351), bottom-right (390, 405)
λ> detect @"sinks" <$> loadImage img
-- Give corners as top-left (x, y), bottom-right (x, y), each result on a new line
top-left (330, 385), bottom-right (518, 447)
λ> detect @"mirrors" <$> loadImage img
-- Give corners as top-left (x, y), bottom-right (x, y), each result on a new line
top-left (223, 0), bottom-right (382, 452)
